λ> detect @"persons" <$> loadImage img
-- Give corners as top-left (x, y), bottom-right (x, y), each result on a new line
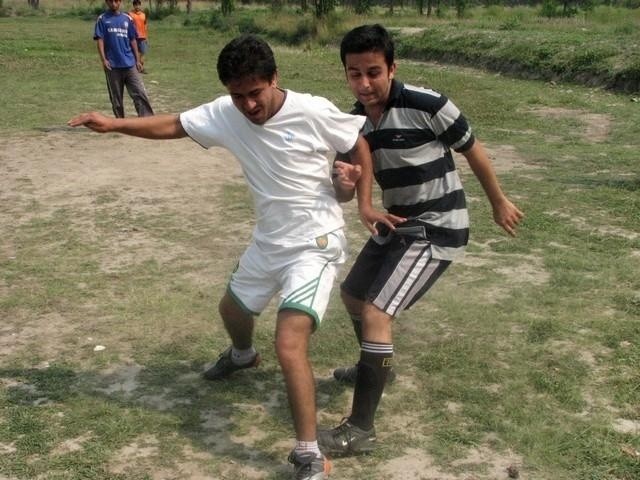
top-left (68, 34), bottom-right (409, 480)
top-left (316, 23), bottom-right (527, 464)
top-left (92, 0), bottom-right (156, 120)
top-left (122, 1), bottom-right (149, 74)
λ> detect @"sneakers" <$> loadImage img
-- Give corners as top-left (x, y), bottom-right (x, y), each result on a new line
top-left (316, 420), bottom-right (378, 456)
top-left (289, 445), bottom-right (331, 480)
top-left (203, 349), bottom-right (262, 380)
top-left (333, 365), bottom-right (396, 384)
